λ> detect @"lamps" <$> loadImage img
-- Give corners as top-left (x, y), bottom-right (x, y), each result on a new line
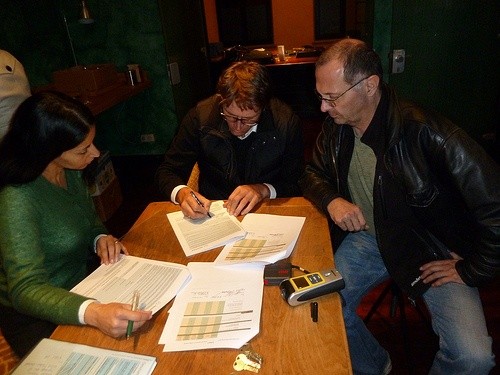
top-left (62, 0), bottom-right (94, 70)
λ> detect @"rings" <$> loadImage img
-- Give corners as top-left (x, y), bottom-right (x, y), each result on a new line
top-left (114, 240), bottom-right (119, 243)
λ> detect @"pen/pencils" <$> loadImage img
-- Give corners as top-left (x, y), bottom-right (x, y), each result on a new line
top-left (127, 290), bottom-right (140, 338)
top-left (190, 191), bottom-right (211, 217)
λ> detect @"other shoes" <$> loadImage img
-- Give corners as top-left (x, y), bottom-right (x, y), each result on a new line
top-left (381, 351), bottom-right (392, 375)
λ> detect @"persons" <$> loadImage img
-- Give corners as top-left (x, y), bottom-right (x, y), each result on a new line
top-left (300, 39), bottom-right (500, 375)
top-left (0, 90), bottom-right (155, 353)
top-left (157, 61), bottom-right (304, 219)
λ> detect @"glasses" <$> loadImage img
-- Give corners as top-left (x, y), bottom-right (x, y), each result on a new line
top-left (314, 73), bottom-right (375, 108)
top-left (220, 106), bottom-right (263, 127)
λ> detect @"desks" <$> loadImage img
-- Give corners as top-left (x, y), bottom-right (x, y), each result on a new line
top-left (49, 196), bottom-right (354, 375)
